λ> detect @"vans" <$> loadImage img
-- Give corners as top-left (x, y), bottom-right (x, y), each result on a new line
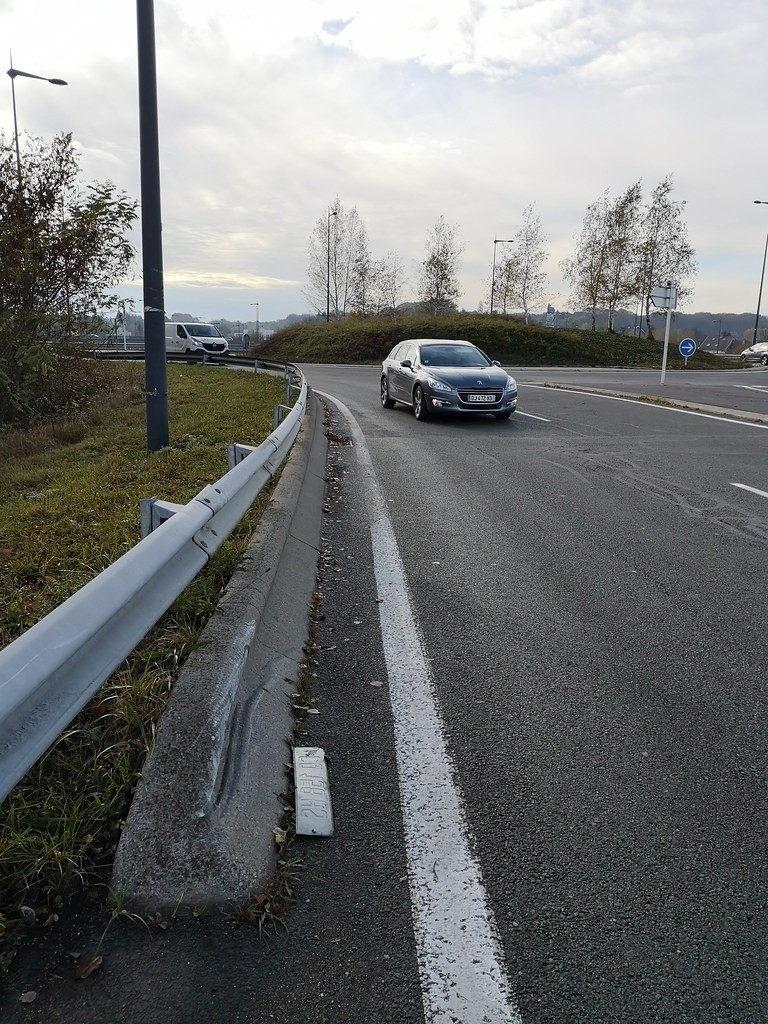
top-left (165, 322), bottom-right (233, 364)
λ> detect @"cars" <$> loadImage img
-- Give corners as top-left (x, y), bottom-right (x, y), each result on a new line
top-left (379, 338), bottom-right (518, 421)
top-left (741, 342), bottom-right (768, 366)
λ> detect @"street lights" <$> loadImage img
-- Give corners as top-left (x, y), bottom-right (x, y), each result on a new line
top-left (327, 212), bottom-right (339, 321)
top-left (713, 319), bottom-right (721, 354)
top-left (6, 48), bottom-right (67, 194)
top-left (250, 302), bottom-right (259, 342)
top-left (491, 240), bottom-right (514, 314)
top-left (752, 200), bottom-right (768, 346)
top-left (119, 300), bottom-right (134, 351)
